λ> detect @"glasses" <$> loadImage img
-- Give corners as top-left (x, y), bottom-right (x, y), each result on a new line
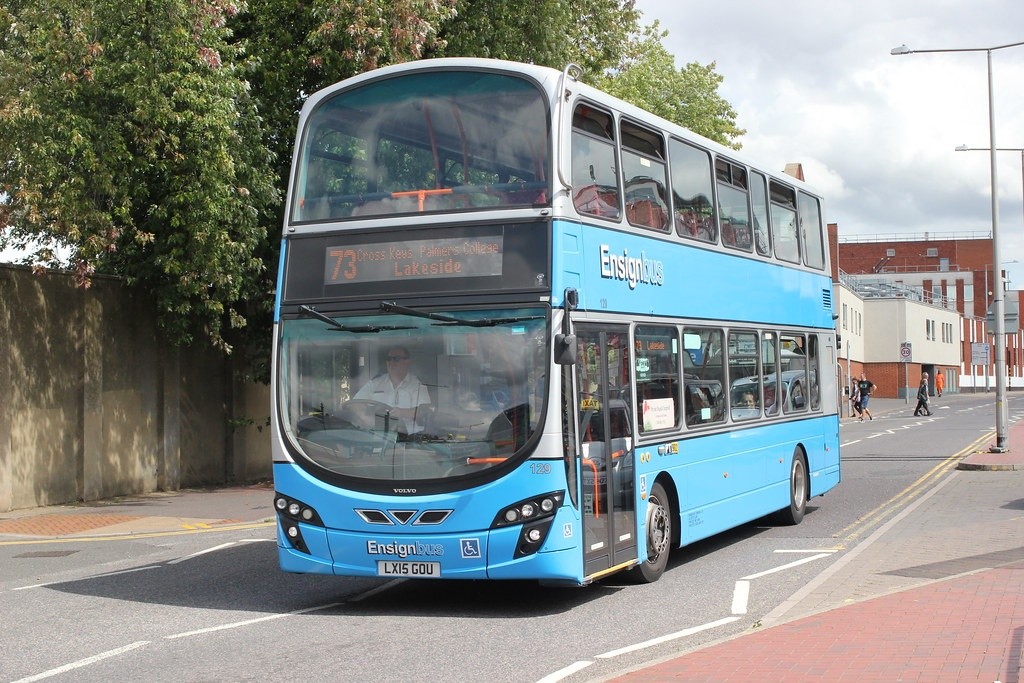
top-left (385, 356), bottom-right (408, 362)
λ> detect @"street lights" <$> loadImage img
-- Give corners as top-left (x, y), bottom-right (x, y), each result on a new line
top-left (954, 145), bottom-right (1024, 153)
top-left (984, 259), bottom-right (1019, 392)
top-left (889, 41), bottom-right (1023, 452)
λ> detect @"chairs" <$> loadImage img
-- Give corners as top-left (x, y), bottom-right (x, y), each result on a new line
top-left (572, 182), bottom-right (751, 250)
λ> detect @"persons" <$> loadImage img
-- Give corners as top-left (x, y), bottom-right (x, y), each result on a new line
top-left (935, 369), bottom-right (944, 397)
top-left (850, 378), bottom-right (862, 417)
top-left (914, 372), bottom-right (933, 416)
top-left (858, 373), bottom-right (878, 421)
top-left (736, 392), bottom-right (755, 409)
top-left (352, 346), bottom-right (432, 438)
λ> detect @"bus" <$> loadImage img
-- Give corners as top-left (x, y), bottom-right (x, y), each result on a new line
top-left (269, 56), bottom-right (841, 590)
